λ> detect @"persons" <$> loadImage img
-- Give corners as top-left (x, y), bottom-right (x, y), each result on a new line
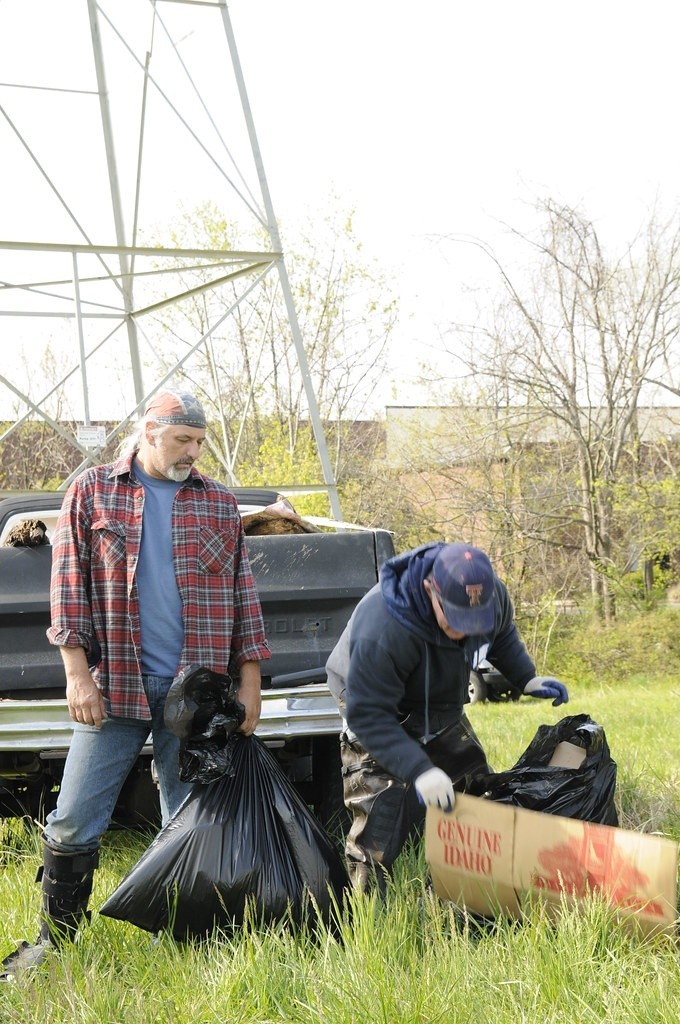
top-left (0, 390), bottom-right (272, 981)
top-left (324, 541), bottom-right (569, 904)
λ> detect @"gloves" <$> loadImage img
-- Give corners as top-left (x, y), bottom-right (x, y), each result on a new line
top-left (413, 765), bottom-right (456, 814)
top-left (525, 676), bottom-right (568, 708)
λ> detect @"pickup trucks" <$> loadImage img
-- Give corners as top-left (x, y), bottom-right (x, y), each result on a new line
top-left (0, 490), bottom-right (398, 833)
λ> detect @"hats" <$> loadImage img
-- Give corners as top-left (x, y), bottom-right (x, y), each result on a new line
top-left (430, 541), bottom-right (498, 639)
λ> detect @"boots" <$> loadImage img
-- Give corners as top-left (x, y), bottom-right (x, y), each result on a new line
top-left (0, 847), bottom-right (100, 986)
